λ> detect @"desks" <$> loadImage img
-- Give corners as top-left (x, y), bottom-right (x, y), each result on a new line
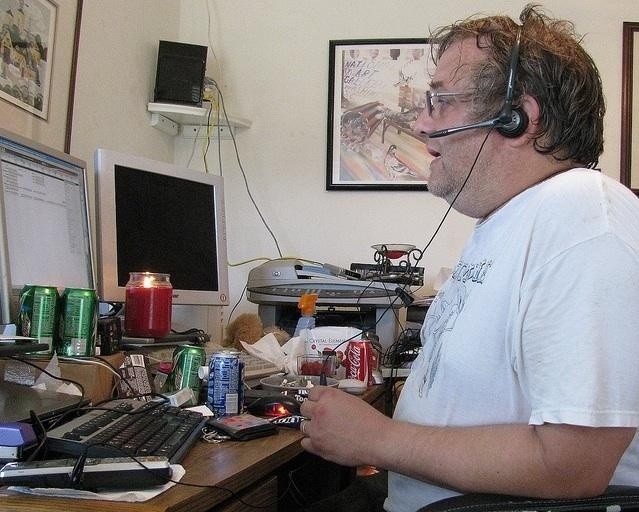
top-left (0, 381), bottom-right (387, 512)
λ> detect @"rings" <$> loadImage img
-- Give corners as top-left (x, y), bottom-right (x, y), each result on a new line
top-left (300, 421), bottom-right (307, 436)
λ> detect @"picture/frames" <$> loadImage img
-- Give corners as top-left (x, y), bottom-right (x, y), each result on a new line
top-left (326, 38), bottom-right (440, 192)
top-left (0, 0), bottom-right (84, 155)
top-left (620, 21), bottom-right (638, 198)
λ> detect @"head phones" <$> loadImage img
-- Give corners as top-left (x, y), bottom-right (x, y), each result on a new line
top-left (429, 23), bottom-right (529, 139)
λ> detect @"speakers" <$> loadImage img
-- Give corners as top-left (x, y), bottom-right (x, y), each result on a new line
top-left (154, 39), bottom-right (208, 107)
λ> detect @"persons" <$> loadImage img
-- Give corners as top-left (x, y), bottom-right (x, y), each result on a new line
top-left (297, 6), bottom-right (639, 511)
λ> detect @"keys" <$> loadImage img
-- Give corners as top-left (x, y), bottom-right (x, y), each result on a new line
top-left (201, 411), bottom-right (275, 443)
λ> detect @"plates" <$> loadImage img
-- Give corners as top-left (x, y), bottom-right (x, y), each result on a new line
top-left (259, 374), bottom-right (341, 391)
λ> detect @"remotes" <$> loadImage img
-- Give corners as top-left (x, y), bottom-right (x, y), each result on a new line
top-left (0, 456), bottom-right (173, 492)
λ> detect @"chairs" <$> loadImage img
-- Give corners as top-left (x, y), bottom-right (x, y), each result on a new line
top-left (416, 484), bottom-right (639, 512)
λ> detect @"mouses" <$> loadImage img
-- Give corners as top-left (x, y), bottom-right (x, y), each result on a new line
top-left (246, 395), bottom-right (302, 418)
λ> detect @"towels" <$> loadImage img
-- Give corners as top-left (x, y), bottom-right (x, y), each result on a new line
top-left (241, 332), bottom-right (306, 373)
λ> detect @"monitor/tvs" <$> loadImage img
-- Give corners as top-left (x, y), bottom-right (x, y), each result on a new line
top-left (95, 148), bottom-right (230, 344)
top-left (0, 127), bottom-right (95, 296)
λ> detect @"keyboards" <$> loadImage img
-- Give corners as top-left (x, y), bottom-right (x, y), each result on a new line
top-left (48, 399), bottom-right (210, 465)
top-left (200, 348), bottom-right (282, 381)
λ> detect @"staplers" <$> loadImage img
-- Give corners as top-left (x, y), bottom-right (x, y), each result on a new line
top-left (0, 422), bottom-right (38, 465)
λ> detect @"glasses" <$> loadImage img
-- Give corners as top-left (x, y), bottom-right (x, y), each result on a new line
top-left (427, 91), bottom-right (478, 115)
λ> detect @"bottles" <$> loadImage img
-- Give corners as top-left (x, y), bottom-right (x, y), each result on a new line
top-left (345, 339), bottom-right (372, 395)
top-left (59, 288), bottom-right (95, 357)
top-left (207, 354), bottom-right (245, 416)
top-left (125, 271), bottom-right (172, 338)
top-left (170, 345), bottom-right (206, 404)
top-left (323, 350), bottom-right (337, 378)
top-left (96, 316), bottom-right (122, 356)
top-left (17, 283), bottom-right (59, 359)
